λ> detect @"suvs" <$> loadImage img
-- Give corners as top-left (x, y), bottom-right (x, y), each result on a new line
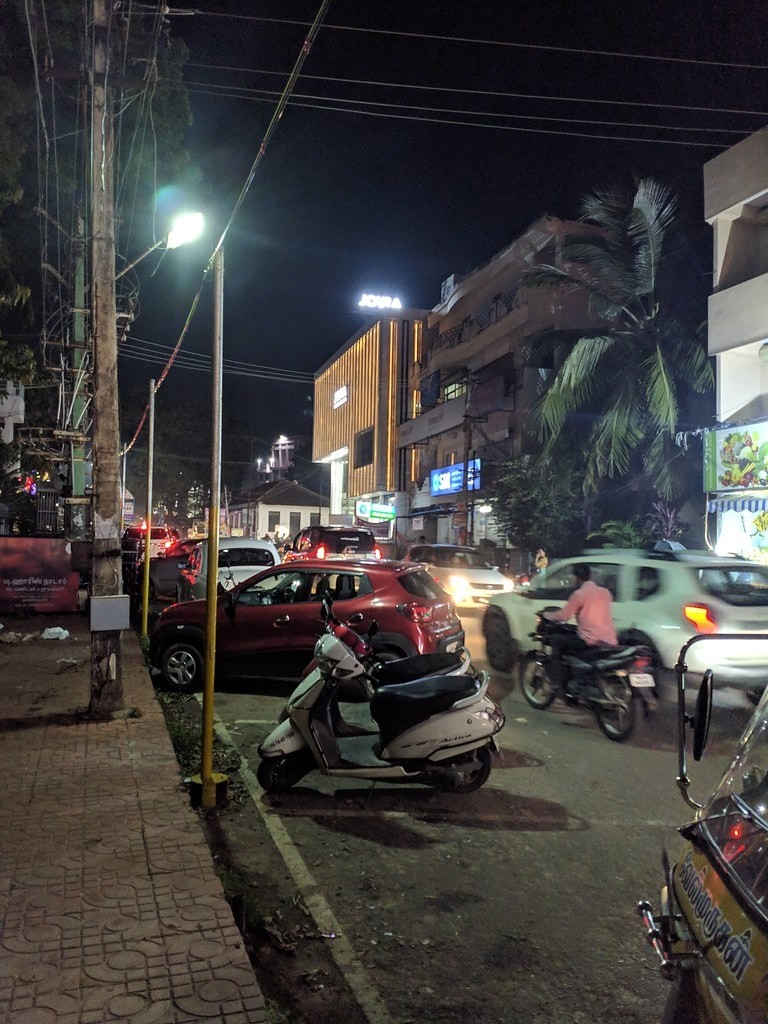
top-left (486, 550), bottom-right (768, 700)
top-left (637, 627), bottom-right (768, 1024)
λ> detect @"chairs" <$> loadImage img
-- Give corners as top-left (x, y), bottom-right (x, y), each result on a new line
top-left (312, 579), bottom-right (334, 602)
top-left (334, 574), bottom-right (352, 599)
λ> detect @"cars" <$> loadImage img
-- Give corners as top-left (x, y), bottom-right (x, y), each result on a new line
top-left (151, 555), bottom-right (466, 701)
top-left (404, 542), bottom-right (512, 614)
top-left (117, 511), bottom-right (380, 604)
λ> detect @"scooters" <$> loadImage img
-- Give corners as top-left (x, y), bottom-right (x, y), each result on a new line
top-left (254, 593), bottom-right (505, 796)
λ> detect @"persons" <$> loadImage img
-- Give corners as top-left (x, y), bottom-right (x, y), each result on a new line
top-left (535, 549), bottom-right (548, 573)
top-left (334, 542), bottom-right (347, 556)
top-left (417, 537), bottom-right (428, 544)
top-left (538, 564), bottom-right (618, 696)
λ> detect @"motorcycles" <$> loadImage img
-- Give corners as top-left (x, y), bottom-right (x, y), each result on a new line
top-left (518, 605), bottom-right (657, 740)
